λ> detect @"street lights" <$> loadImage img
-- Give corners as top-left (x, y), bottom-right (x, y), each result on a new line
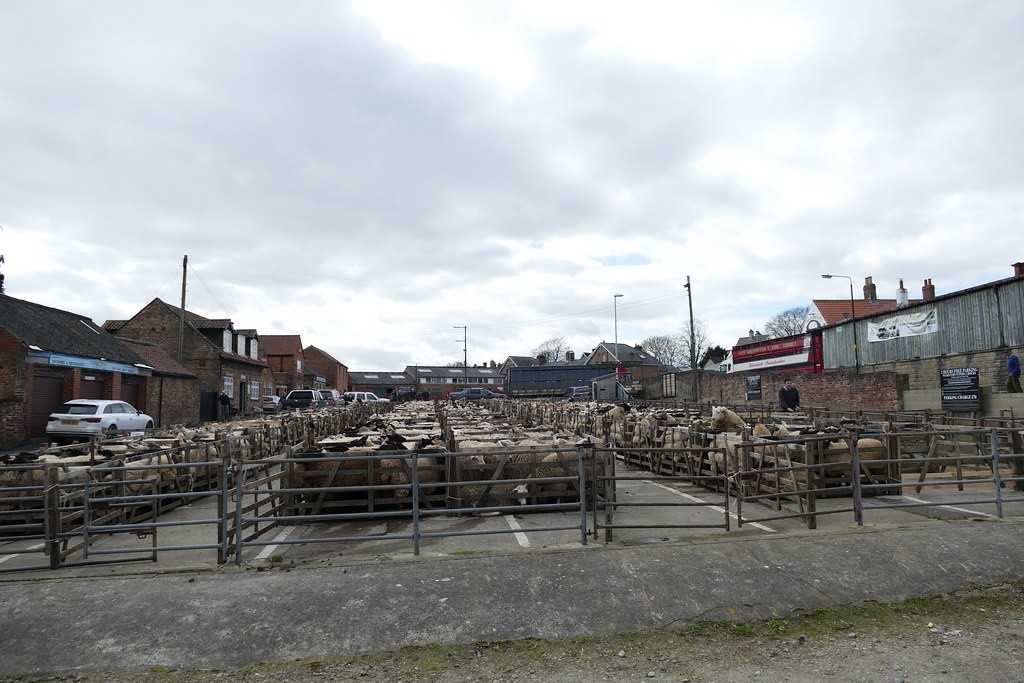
top-left (614, 294), bottom-right (623, 381)
top-left (806, 320), bottom-right (822, 373)
top-left (821, 274), bottom-right (858, 374)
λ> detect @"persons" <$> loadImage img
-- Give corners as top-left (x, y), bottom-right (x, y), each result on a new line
top-left (1005, 349), bottom-right (1024, 393)
top-left (779, 379), bottom-right (800, 411)
top-left (218, 390), bottom-right (231, 423)
top-left (278, 391), bottom-right (286, 408)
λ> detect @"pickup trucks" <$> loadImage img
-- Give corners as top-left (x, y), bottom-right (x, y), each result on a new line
top-left (449, 387), bottom-right (508, 401)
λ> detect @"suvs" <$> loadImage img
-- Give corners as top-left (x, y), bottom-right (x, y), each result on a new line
top-left (262, 389), bottom-right (392, 415)
top-left (45, 398), bottom-right (156, 445)
top-left (394, 386), bottom-right (430, 398)
top-left (563, 386), bottom-right (591, 401)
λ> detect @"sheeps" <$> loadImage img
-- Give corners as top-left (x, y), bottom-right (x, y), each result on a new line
top-left (0, 398), bottom-right (885, 539)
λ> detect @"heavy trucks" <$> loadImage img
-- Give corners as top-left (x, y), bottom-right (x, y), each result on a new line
top-left (718, 329), bottom-right (824, 374)
top-left (504, 363), bottom-right (632, 400)
